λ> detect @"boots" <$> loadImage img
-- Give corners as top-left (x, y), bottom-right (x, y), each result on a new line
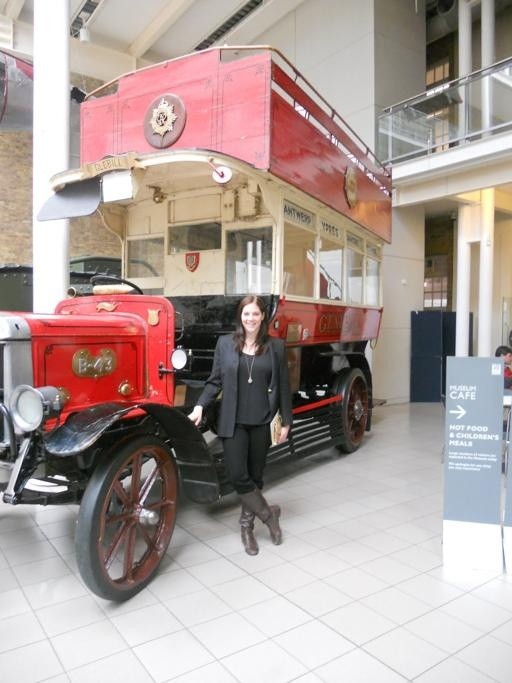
top-left (231, 482), bottom-right (282, 555)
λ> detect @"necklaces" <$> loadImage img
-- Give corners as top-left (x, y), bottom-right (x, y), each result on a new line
top-left (242, 342), bottom-right (259, 384)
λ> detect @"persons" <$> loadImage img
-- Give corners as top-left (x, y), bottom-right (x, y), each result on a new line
top-left (495, 345), bottom-right (512, 389)
top-left (186, 295), bottom-right (293, 556)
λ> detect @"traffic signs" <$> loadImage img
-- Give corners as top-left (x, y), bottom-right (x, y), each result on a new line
top-left (444, 355), bottom-right (502, 525)
